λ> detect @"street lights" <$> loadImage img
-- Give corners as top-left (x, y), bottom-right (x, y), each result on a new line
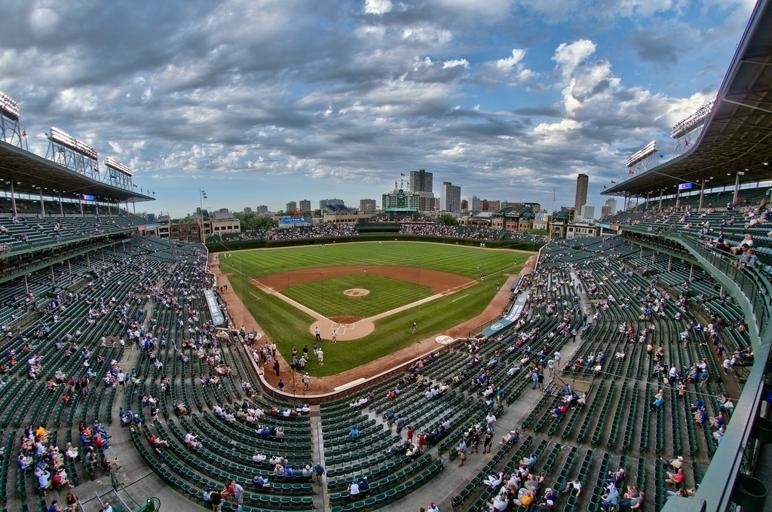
top-left (198, 187), bottom-right (209, 246)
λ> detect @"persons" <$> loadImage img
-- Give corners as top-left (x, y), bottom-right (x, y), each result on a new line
top-left (504, 195), bottom-right (770, 510)
top-left (497, 280), bottom-right (500, 290)
top-left (2, 198), bottom-right (269, 510)
top-left (269, 321), bottom-right (518, 510)
top-left (480, 272), bottom-right (484, 280)
top-left (266, 215), bottom-right (550, 248)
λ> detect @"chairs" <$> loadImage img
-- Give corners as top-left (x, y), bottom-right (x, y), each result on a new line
top-left (223, 320), bottom-right (323, 512)
top-left (224, 225), bottom-right (322, 251)
top-left (324, 319), bottom-right (772, 512)
top-left (0, 190), bottom-right (239, 512)
top-left (503, 186), bottom-right (772, 319)
top-left (324, 213), bottom-right (503, 243)
